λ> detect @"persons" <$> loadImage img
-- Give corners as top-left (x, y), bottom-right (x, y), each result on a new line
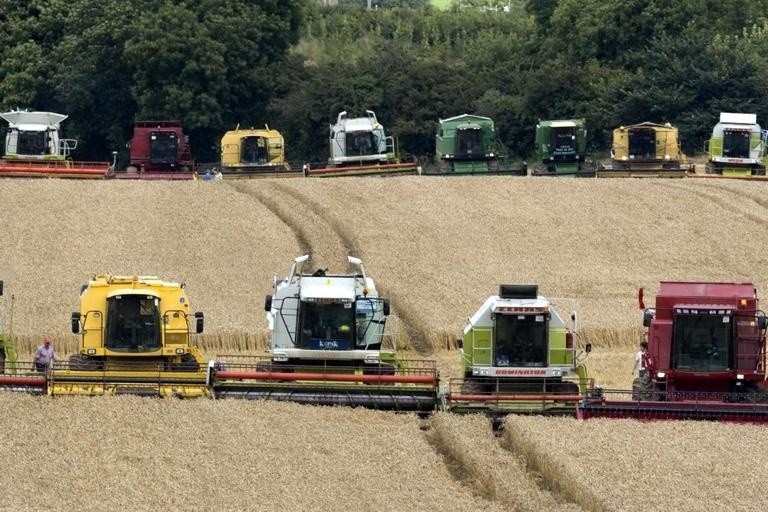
top-left (31, 338), bottom-right (58, 373)
top-left (630, 342), bottom-right (648, 378)
top-left (203, 168), bottom-right (212, 180)
top-left (213, 169), bottom-right (222, 181)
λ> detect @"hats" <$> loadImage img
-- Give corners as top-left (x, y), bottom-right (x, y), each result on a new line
top-left (44, 336), bottom-right (52, 343)
